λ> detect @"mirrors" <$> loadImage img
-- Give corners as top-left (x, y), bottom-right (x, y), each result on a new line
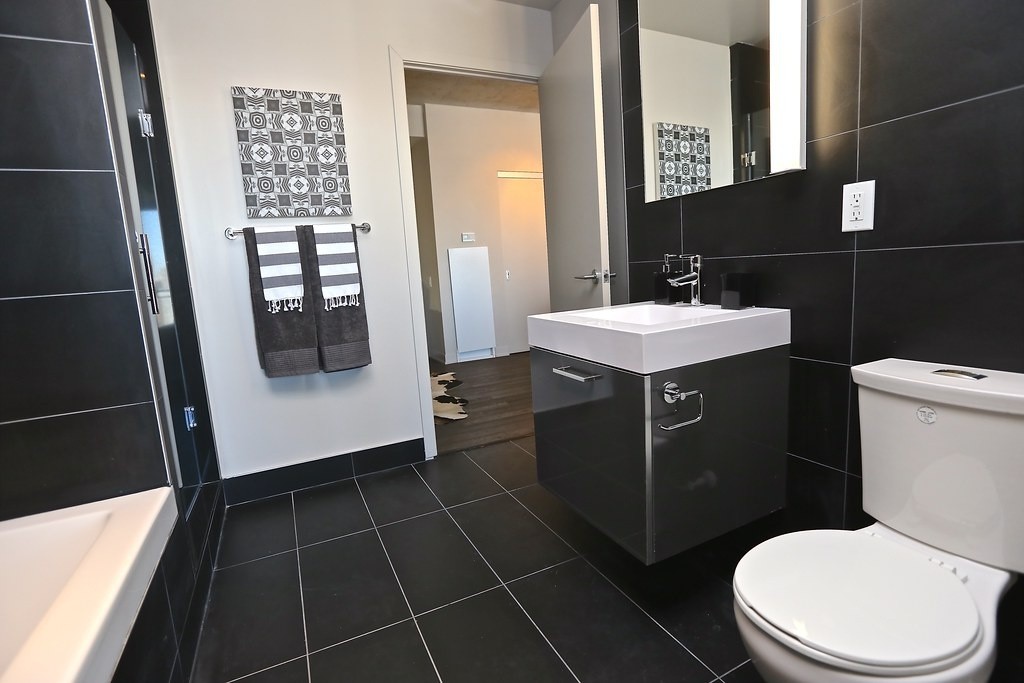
top-left (637, 0), bottom-right (807, 202)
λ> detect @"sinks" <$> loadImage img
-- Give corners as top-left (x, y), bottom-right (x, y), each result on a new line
top-left (1, 482), bottom-right (180, 683)
top-left (526, 299), bottom-right (792, 376)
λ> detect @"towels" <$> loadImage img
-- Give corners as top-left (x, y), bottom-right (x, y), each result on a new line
top-left (241, 222), bottom-right (373, 379)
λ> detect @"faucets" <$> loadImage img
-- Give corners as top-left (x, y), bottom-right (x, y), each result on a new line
top-left (666, 254), bottom-right (702, 307)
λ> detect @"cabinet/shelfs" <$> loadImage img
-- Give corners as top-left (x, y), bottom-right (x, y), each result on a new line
top-left (531, 344), bottom-right (792, 567)
top-left (0, 0), bottom-right (216, 521)
top-left (729, 40), bottom-right (771, 183)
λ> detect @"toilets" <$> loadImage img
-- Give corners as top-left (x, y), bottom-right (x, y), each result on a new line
top-left (732, 354), bottom-right (1023, 683)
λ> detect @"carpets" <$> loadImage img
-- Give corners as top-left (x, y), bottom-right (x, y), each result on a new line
top-left (430, 369), bottom-right (470, 420)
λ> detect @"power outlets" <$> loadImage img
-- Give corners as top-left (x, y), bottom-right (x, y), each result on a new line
top-left (841, 179), bottom-right (876, 232)
